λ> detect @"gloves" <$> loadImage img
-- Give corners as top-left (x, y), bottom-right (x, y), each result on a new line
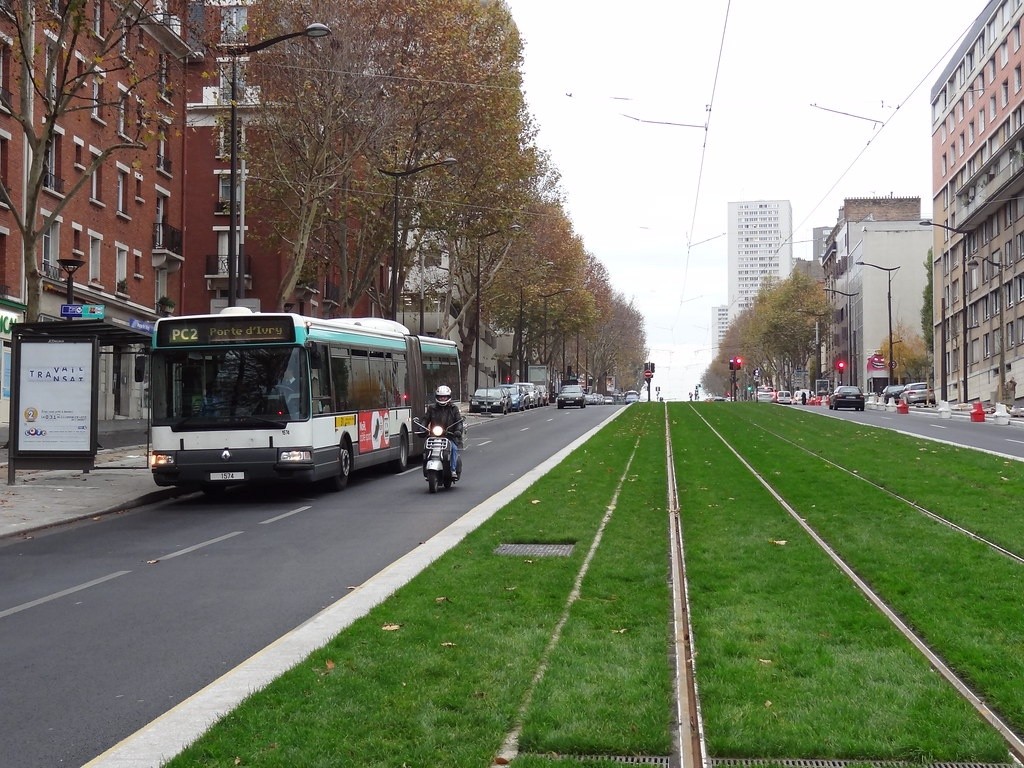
top-left (452, 430), bottom-right (461, 438)
top-left (418, 428), bottom-right (428, 438)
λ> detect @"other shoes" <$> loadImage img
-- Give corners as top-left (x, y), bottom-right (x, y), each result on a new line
top-left (452, 471), bottom-right (457, 477)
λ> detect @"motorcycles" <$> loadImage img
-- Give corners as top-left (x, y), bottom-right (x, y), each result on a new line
top-left (412, 416), bottom-right (468, 495)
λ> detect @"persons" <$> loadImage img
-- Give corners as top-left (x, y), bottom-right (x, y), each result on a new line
top-left (801, 392), bottom-right (806, 405)
top-left (271, 364), bottom-right (297, 394)
top-left (1006, 377), bottom-right (1017, 396)
top-left (418, 385), bottom-right (463, 479)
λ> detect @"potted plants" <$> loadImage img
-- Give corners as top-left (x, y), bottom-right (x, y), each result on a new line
top-left (159, 297), bottom-right (175, 312)
top-left (118, 278), bottom-right (128, 294)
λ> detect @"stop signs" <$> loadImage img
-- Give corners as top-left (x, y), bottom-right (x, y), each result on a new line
top-left (643, 370), bottom-right (652, 378)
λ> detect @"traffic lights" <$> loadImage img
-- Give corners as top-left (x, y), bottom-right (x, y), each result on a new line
top-left (651, 363), bottom-right (656, 373)
top-left (838, 361), bottom-right (844, 374)
top-left (735, 357), bottom-right (742, 370)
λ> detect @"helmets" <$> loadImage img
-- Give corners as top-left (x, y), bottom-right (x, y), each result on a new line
top-left (435, 386), bottom-right (451, 406)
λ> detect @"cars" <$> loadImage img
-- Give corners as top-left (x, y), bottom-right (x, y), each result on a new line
top-left (881, 381), bottom-right (937, 407)
top-left (470, 382), bottom-right (817, 418)
top-left (825, 386), bottom-right (864, 412)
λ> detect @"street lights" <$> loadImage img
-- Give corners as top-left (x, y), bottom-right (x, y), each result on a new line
top-left (475, 224), bottom-right (522, 392)
top-left (390, 157), bottom-right (459, 318)
top-left (855, 262), bottom-right (894, 385)
top-left (919, 220), bottom-right (966, 403)
top-left (797, 309), bottom-right (822, 380)
top-left (823, 287), bottom-right (853, 387)
top-left (543, 287), bottom-right (573, 364)
top-left (228, 24), bottom-right (334, 305)
top-left (969, 255), bottom-right (1006, 404)
top-left (58, 257), bottom-right (88, 320)
top-left (518, 262), bottom-right (554, 382)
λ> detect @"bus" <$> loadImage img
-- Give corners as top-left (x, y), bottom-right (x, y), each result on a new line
top-left (133, 306), bottom-right (461, 500)
